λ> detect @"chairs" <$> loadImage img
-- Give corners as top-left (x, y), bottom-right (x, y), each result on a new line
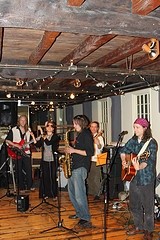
top-left (31, 152), bottom-right (42, 180)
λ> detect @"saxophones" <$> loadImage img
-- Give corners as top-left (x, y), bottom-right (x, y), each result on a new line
top-left (58, 128), bottom-right (75, 179)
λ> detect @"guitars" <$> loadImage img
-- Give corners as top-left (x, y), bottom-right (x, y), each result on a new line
top-left (121, 151), bottom-right (149, 182)
top-left (8, 135), bottom-right (42, 160)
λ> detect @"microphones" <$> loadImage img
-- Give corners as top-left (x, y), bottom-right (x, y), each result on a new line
top-left (15, 146), bottom-right (26, 157)
top-left (119, 131), bottom-right (128, 136)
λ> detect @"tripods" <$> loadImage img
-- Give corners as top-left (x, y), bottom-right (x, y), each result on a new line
top-left (30, 150), bottom-right (59, 212)
top-left (40, 153), bottom-right (79, 234)
top-left (0, 142), bottom-right (18, 199)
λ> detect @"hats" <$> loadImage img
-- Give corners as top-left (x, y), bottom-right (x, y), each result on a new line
top-left (134, 118), bottom-right (149, 126)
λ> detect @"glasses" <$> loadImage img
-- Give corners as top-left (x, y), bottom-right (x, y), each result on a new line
top-left (47, 124), bottom-right (54, 127)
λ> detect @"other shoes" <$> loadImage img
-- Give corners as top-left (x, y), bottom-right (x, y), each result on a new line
top-left (94, 195), bottom-right (100, 201)
top-left (53, 197), bottom-right (57, 201)
top-left (69, 214), bottom-right (77, 217)
top-left (44, 197), bottom-right (48, 200)
top-left (20, 190), bottom-right (29, 193)
top-left (78, 220), bottom-right (89, 225)
top-left (126, 228), bottom-right (153, 239)
top-left (31, 188), bottom-right (36, 191)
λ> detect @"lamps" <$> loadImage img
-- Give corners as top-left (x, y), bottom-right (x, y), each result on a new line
top-left (74, 80), bottom-right (81, 87)
top-left (68, 62), bottom-right (77, 72)
top-left (70, 92), bottom-right (75, 99)
top-left (16, 78), bottom-right (24, 86)
top-left (142, 38), bottom-right (159, 60)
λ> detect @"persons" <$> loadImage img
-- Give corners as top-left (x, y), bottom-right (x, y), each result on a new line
top-left (118, 118), bottom-right (158, 240)
top-left (5, 115), bottom-right (40, 193)
top-left (30, 122), bottom-right (60, 201)
top-left (60, 115), bottom-right (95, 228)
top-left (87, 121), bottom-right (104, 200)
